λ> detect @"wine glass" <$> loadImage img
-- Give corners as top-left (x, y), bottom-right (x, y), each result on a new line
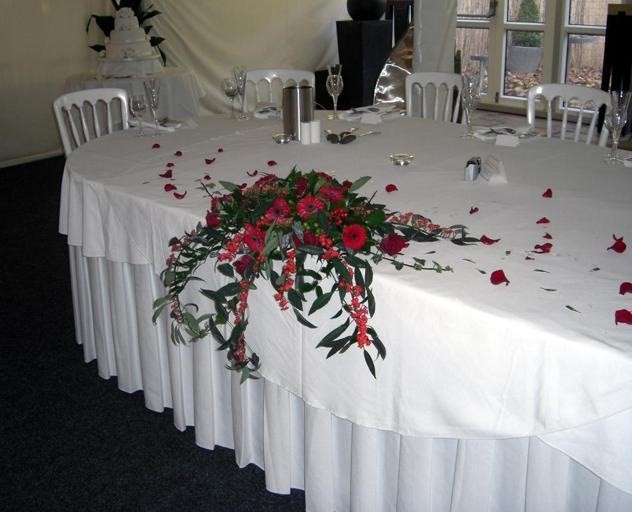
top-left (222, 65), bottom-right (248, 119)
top-left (460, 75), bottom-right (480, 140)
top-left (325, 63), bottom-right (345, 121)
top-left (129, 77), bottom-right (167, 137)
top-left (604, 89), bottom-right (632, 163)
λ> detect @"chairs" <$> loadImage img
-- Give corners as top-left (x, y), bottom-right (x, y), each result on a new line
top-left (405, 72), bottom-right (469, 124)
top-left (239, 69), bottom-right (316, 111)
top-left (527, 84), bottom-right (613, 146)
top-left (53, 87), bottom-right (130, 155)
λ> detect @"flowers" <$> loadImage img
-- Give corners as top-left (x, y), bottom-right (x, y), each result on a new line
top-left (149, 144), bottom-right (632, 383)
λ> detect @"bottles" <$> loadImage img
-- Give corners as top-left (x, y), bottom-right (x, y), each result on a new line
top-left (302, 119), bottom-right (320, 144)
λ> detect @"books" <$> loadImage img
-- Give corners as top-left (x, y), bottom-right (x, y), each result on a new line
top-left (339, 100), bottom-right (408, 121)
